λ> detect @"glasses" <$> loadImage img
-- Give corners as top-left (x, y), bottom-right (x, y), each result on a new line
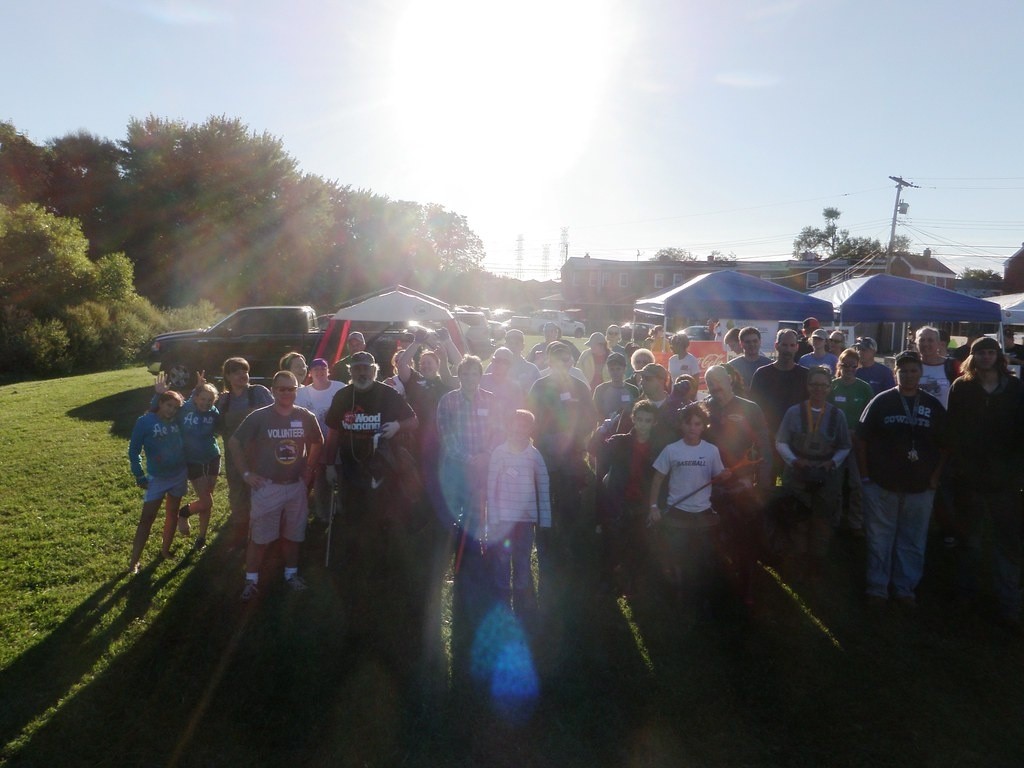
top-left (310, 368), bottom-right (327, 371)
top-left (275, 386), bottom-right (297, 392)
top-left (491, 357), bottom-right (512, 364)
top-left (607, 331), bottom-right (619, 335)
top-left (293, 365), bottom-right (308, 369)
top-left (828, 338), bottom-right (844, 343)
top-left (839, 362), bottom-right (858, 368)
top-left (808, 383), bottom-right (830, 388)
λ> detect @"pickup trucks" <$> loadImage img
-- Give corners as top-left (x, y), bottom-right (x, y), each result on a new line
top-left (511, 310), bottom-right (585, 338)
top-left (146, 307), bottom-right (417, 388)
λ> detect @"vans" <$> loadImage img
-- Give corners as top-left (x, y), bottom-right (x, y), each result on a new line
top-left (451, 312), bottom-right (491, 350)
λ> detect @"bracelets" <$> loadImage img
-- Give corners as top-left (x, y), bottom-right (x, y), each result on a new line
top-left (304, 464), bottom-right (315, 472)
top-left (243, 472), bottom-right (250, 479)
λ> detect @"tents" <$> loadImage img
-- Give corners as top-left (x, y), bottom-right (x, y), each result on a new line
top-left (808, 273), bottom-right (1005, 352)
top-left (982, 293), bottom-right (1024, 326)
top-left (631, 271), bottom-right (833, 353)
top-left (334, 285), bottom-right (452, 325)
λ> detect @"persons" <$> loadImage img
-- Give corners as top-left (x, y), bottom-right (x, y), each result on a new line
top-left (213, 357), bottom-right (279, 558)
top-left (147, 371), bottom-right (222, 554)
top-left (275, 318), bottom-right (1024, 609)
top-left (227, 370), bottom-right (325, 608)
top-left (127, 369), bottom-right (210, 574)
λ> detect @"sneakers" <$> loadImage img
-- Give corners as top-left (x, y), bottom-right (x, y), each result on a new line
top-left (237, 579), bottom-right (261, 604)
top-left (285, 573), bottom-right (311, 594)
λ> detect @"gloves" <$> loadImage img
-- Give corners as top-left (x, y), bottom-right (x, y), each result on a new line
top-left (600, 411), bottom-right (620, 432)
top-left (414, 329), bottom-right (429, 343)
top-left (434, 327), bottom-right (450, 342)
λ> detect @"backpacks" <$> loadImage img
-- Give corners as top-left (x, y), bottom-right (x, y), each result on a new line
top-left (790, 400), bottom-right (839, 486)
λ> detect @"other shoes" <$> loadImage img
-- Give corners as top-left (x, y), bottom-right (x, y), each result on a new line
top-left (943, 536), bottom-right (960, 548)
top-left (896, 596), bottom-right (920, 611)
top-left (861, 592), bottom-right (885, 604)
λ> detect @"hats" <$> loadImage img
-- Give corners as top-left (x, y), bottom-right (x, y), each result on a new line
top-left (971, 337), bottom-right (1002, 351)
top-left (802, 317), bottom-right (821, 328)
top-left (895, 350), bottom-right (921, 364)
top-left (347, 331), bottom-right (364, 342)
top-left (851, 337), bottom-right (878, 352)
top-left (350, 351), bottom-right (375, 367)
top-left (584, 332), bottom-right (606, 345)
top-left (607, 353), bottom-right (626, 364)
top-left (634, 363), bottom-right (667, 379)
top-left (309, 359), bottom-right (328, 368)
top-left (810, 329), bottom-right (828, 340)
